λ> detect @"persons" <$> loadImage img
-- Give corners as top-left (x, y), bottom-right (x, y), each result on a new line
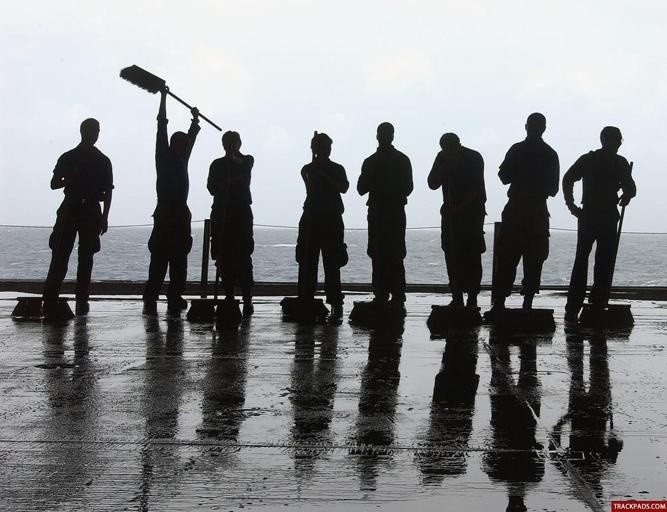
top-left (295, 133), bottom-right (350, 316)
top-left (562, 126), bottom-right (638, 321)
top-left (483, 112), bottom-right (560, 324)
top-left (43, 118), bottom-right (114, 316)
top-left (142, 87), bottom-right (201, 315)
top-left (427, 132), bottom-right (487, 307)
top-left (207, 130), bottom-right (255, 315)
top-left (356, 122), bottom-right (414, 317)
